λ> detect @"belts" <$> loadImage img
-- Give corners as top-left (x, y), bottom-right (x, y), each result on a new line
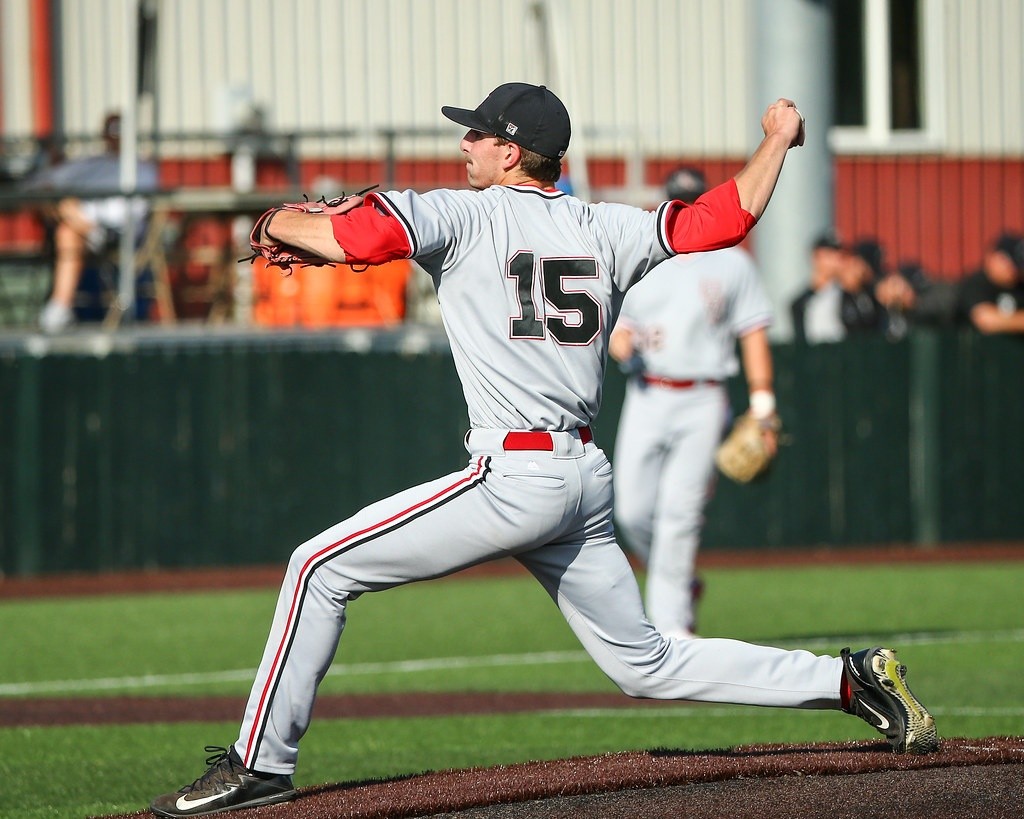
top-left (644, 378), bottom-right (723, 391)
top-left (503, 425), bottom-right (592, 451)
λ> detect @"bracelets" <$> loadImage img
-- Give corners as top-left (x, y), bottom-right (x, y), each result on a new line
top-left (264, 207), bottom-right (285, 244)
top-left (751, 389), bottom-right (777, 420)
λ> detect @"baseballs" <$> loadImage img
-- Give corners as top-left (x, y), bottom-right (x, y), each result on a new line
top-left (789, 106), bottom-right (806, 133)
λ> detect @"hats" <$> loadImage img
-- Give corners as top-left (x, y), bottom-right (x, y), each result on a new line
top-left (810, 228), bottom-right (1024, 295)
top-left (440, 83), bottom-right (572, 159)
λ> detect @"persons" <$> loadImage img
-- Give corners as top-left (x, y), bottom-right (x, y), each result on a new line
top-left (149, 81), bottom-right (936, 819)
top-left (608, 166), bottom-right (782, 642)
top-left (793, 232), bottom-right (1024, 346)
top-left (27, 114), bottom-right (157, 332)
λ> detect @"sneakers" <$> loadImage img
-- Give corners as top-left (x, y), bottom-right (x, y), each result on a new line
top-left (841, 643), bottom-right (937, 756)
top-left (147, 743), bottom-right (298, 818)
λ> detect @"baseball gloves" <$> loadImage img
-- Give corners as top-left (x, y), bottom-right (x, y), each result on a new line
top-left (248, 194), bottom-right (364, 268)
top-left (713, 406), bottom-right (783, 487)
